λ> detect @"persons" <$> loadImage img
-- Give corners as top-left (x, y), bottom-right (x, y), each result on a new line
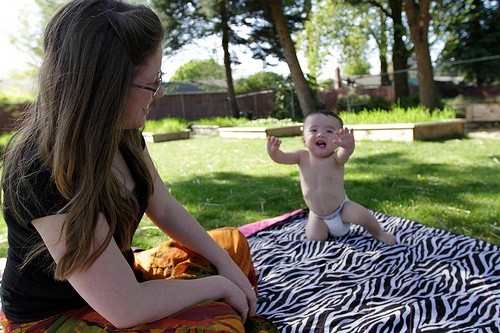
top-left (0, 0), bottom-right (258, 333)
top-left (267, 109), bottom-right (396, 245)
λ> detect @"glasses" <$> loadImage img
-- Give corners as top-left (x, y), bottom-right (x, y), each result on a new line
top-left (130, 70), bottom-right (162, 95)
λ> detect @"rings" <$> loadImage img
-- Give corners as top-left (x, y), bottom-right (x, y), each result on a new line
top-left (251, 287), bottom-right (254, 290)
top-left (246, 307), bottom-right (249, 312)
